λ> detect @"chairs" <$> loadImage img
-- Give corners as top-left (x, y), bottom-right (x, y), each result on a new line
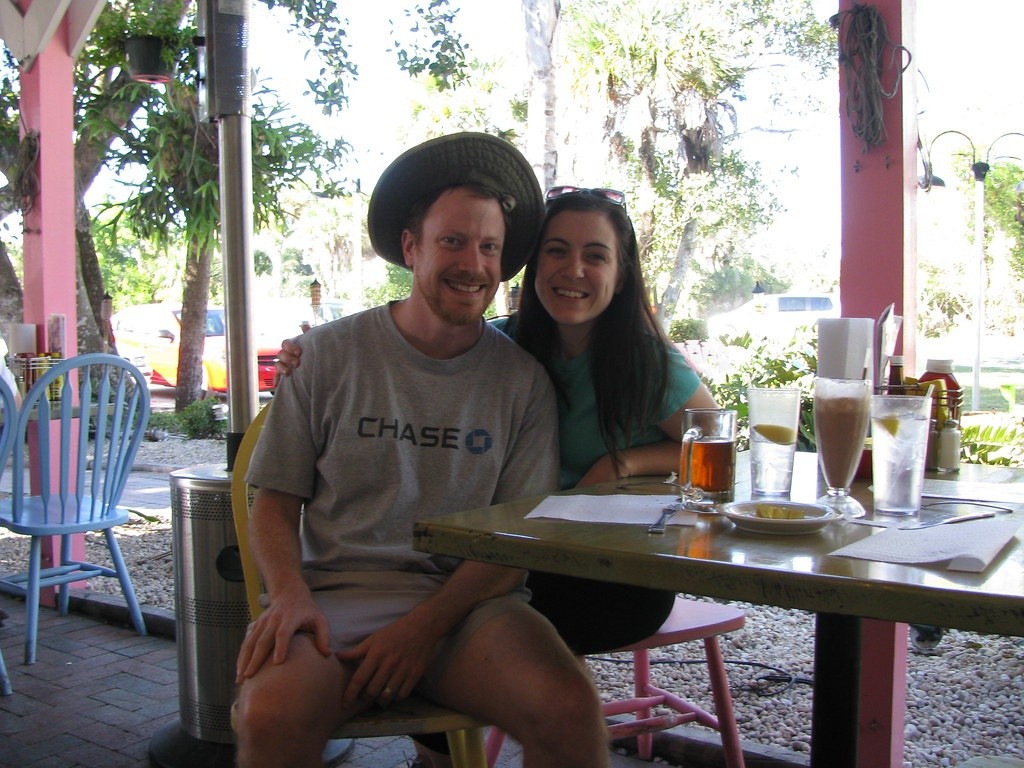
top-left (477, 596), bottom-right (747, 768)
top-left (0, 358), bottom-right (150, 665)
top-left (231, 401), bottom-right (490, 768)
top-left (0, 375), bottom-right (18, 695)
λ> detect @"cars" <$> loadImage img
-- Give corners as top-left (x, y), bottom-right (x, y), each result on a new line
top-left (110, 301), bottom-right (342, 400)
top-left (705, 293), bottom-right (841, 345)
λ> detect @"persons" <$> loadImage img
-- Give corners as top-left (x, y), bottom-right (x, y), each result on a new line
top-left (230, 131), bottom-right (613, 768)
top-left (270, 182), bottom-right (718, 768)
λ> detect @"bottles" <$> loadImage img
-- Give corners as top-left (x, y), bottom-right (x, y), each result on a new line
top-left (926, 419), bottom-right (961, 472)
top-left (13, 351), bottom-right (63, 403)
top-left (919, 358), bottom-right (962, 418)
top-left (888, 356), bottom-right (905, 396)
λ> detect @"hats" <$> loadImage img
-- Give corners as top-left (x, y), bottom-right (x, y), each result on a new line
top-left (368, 131), bottom-right (544, 282)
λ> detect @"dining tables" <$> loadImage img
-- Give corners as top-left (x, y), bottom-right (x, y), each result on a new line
top-left (409, 448), bottom-right (1024, 768)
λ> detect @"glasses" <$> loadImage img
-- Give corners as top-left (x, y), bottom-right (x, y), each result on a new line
top-left (543, 186), bottom-right (626, 215)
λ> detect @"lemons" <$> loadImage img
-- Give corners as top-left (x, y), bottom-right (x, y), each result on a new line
top-left (880, 415), bottom-right (899, 437)
top-left (755, 505), bottom-right (804, 519)
top-left (753, 424), bottom-right (796, 446)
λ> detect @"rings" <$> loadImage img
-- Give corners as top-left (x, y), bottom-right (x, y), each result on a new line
top-left (382, 688), bottom-right (394, 697)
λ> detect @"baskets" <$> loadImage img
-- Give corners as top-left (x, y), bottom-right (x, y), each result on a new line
top-left (870, 385), bottom-right (963, 474)
top-left (10, 357), bottom-right (66, 403)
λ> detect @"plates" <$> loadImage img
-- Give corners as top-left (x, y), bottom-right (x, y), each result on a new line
top-left (718, 500), bottom-right (836, 534)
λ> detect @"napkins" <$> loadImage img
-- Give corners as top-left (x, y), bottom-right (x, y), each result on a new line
top-left (524, 492), bottom-right (680, 527)
top-left (828, 507), bottom-right (1018, 573)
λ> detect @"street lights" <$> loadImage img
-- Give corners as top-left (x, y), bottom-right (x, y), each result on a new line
top-left (751, 281), bottom-right (766, 356)
top-left (917, 130), bottom-right (1024, 411)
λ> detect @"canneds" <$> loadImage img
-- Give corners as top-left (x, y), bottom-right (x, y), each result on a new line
top-left (13, 352), bottom-right (36, 395)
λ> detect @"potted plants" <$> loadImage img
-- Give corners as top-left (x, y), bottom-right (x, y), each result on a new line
top-left (147, 409), bottom-right (181, 443)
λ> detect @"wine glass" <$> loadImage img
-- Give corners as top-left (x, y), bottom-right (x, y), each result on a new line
top-left (813, 376), bottom-right (872, 518)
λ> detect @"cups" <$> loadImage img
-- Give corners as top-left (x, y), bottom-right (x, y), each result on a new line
top-left (747, 388), bottom-right (802, 502)
top-left (679, 408), bottom-right (737, 514)
top-left (871, 395), bottom-right (932, 517)
